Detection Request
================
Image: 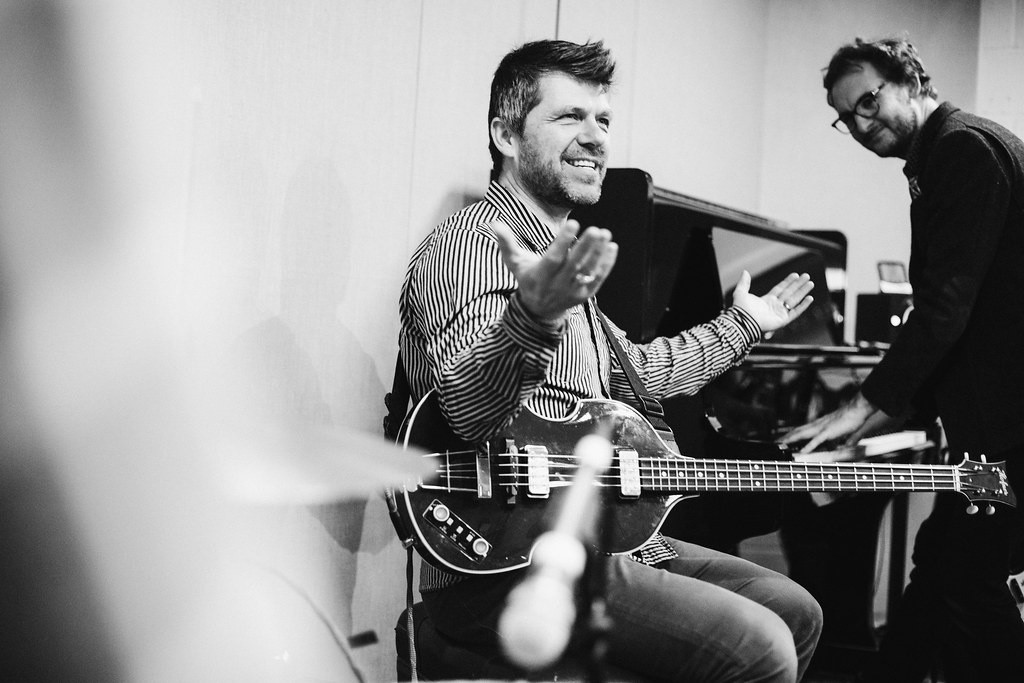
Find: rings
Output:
[575,273,597,285]
[784,302,790,310]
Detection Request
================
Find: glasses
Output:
[831,80,890,135]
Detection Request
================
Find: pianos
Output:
[461,164,946,654]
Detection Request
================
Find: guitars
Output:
[384,383,1019,586]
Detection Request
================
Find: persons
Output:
[772,36,1023,683]
[381,40,824,683]
